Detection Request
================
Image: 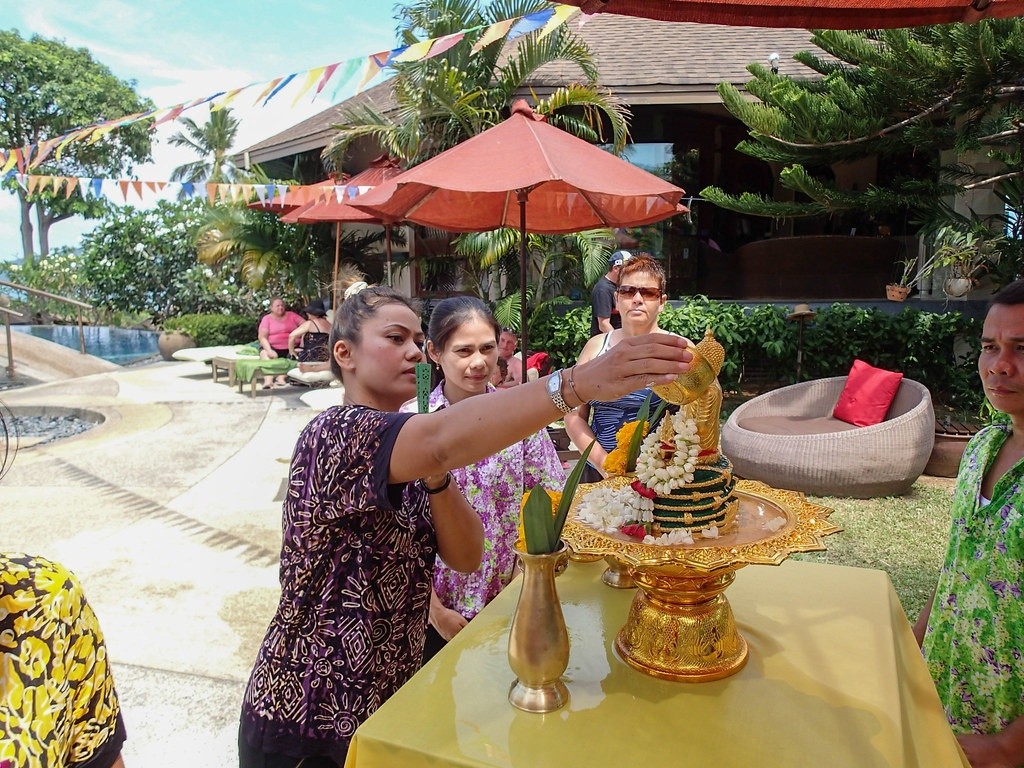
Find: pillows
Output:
[834,358,904,427]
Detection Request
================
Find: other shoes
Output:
[291,381,305,386]
[262,383,273,389]
[276,377,286,386]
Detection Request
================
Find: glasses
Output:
[617,285,662,301]
[502,327,517,335]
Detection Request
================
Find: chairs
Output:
[175,345,550,411]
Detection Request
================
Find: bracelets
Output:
[568,364,588,405]
[420,473,451,494]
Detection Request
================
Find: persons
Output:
[564,253,697,484]
[239,257,694,768]
[422,293,566,668]
[0,397,128,767]
[591,250,633,338]
[911,280,1024,768]
[257,295,307,388]
[490,357,509,387]
[679,322,723,452]
[287,297,333,385]
[498,329,518,360]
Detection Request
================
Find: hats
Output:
[608,251,632,267]
[303,300,327,316]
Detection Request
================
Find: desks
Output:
[344,560,973,768]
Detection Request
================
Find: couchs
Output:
[720,374,936,499]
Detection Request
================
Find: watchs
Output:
[545,367,577,415]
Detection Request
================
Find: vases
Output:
[509,537,571,714]
[599,555,637,588]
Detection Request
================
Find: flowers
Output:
[602,392,669,475]
[508,434,597,553]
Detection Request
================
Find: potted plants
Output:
[886,245,976,301]
[944,227,985,299]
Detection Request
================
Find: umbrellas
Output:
[345,96,691,382]
[247,172,353,352]
[275,150,406,287]
